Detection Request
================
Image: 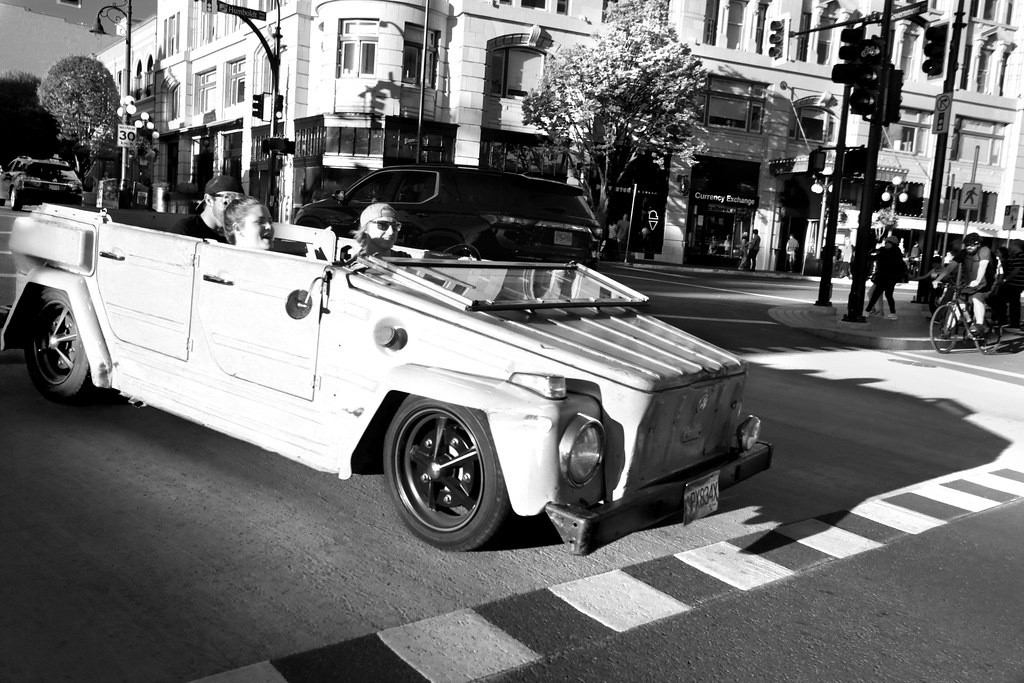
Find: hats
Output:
[360,203,399,229]
[883,236,899,246]
[642,228,650,233]
[196,175,245,210]
[961,233,980,244]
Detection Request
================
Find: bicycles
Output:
[928,283,1002,356]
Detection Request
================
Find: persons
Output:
[345,202,412,268]
[785,234,799,273]
[311,185,326,202]
[862,236,904,320]
[912,233,1024,340]
[737,230,760,272]
[608,214,650,262]
[709,236,718,254]
[172,175,245,245]
[221,194,275,251]
[724,235,732,255]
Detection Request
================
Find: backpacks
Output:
[990,253,1004,295]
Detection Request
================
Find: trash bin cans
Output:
[130,181,149,209]
[153,182,169,213]
[96,178,118,210]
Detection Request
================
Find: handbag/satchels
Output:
[897,261,909,283]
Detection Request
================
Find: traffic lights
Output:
[808,150,826,171]
[252,93,271,122]
[832,25,903,126]
[922,21,949,81]
[767,13,792,66]
[274,96,283,120]
[268,138,295,156]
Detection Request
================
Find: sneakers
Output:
[883,313,898,320]
[862,311,870,317]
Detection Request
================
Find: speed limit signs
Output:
[117,123,136,149]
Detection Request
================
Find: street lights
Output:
[811,167,834,270]
[880,176,909,237]
[89,0,136,191]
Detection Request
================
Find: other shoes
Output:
[870,309,883,316]
[926,316,941,324]
[970,324,986,338]
[937,331,951,339]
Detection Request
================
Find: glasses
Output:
[885,241,890,244]
[370,220,402,231]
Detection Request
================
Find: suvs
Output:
[293,164,603,274]
[0,156,82,211]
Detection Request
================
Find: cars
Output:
[0,203,776,556]
